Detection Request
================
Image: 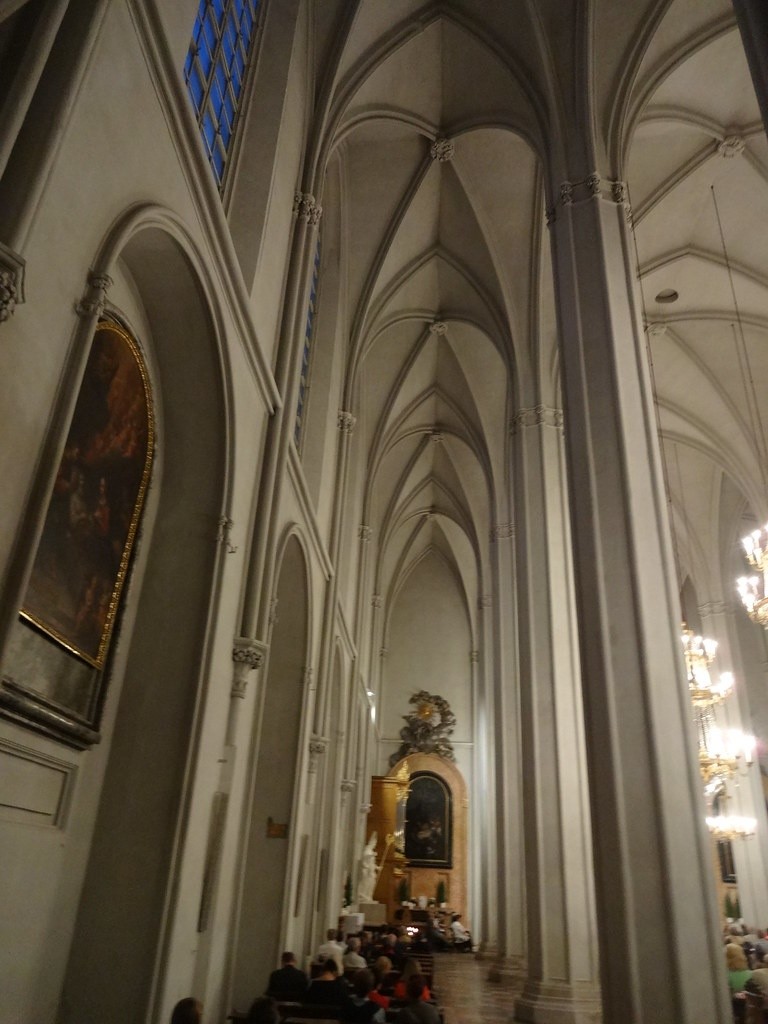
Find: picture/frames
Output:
[17,321,158,669]
[403,776,449,863]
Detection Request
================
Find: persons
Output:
[722,921,768,1024]
[453,914,473,952]
[153,923,441,1024]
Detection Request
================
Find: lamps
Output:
[737,521,768,630]
[681,621,758,844]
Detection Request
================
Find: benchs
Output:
[227,943,445,1024]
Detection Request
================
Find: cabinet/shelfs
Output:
[391,904,448,928]
